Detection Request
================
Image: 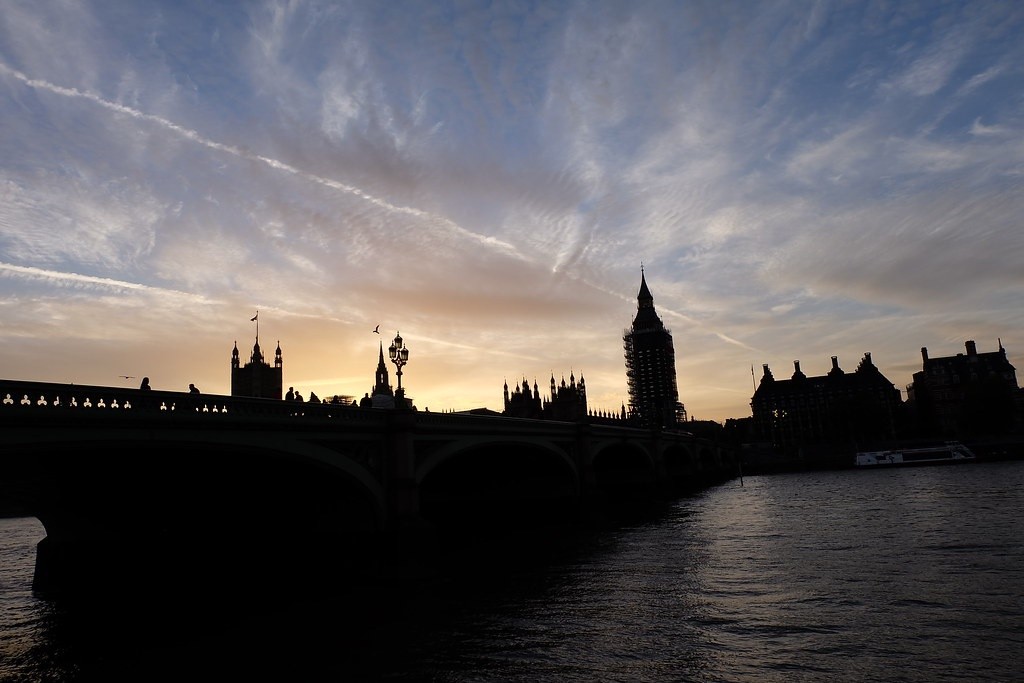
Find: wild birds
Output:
[119,376,134,379]
[373,324,379,334]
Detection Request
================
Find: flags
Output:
[250,315,258,321]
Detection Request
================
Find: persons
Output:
[322,399,328,404]
[285,387,303,416]
[360,393,373,407]
[351,400,358,406]
[330,396,340,404]
[425,407,429,412]
[141,377,151,389]
[190,383,200,394]
[413,406,418,411]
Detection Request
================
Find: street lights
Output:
[389,333,409,401]
